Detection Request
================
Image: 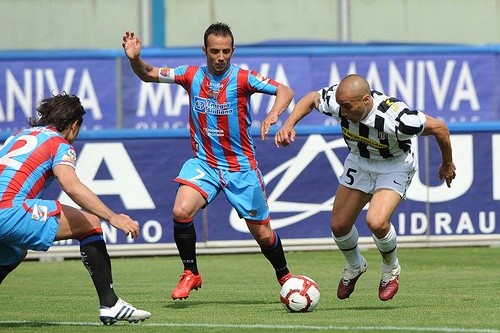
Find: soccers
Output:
[280,275,320,313]
